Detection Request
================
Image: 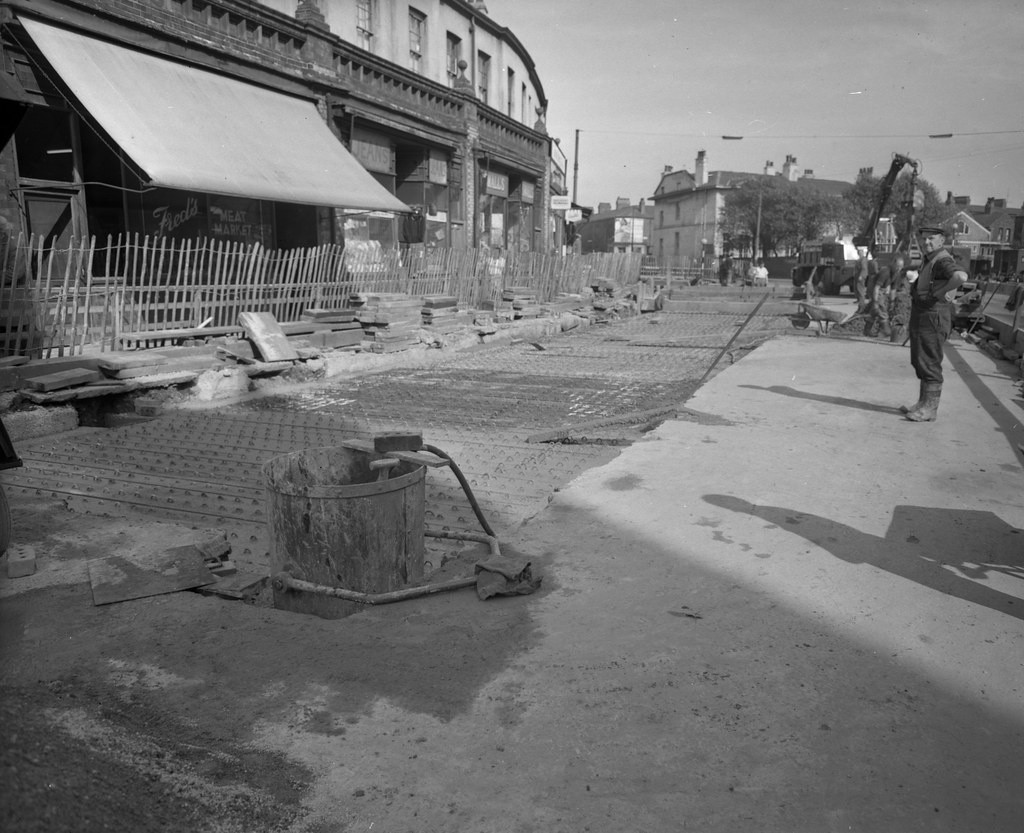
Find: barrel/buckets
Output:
[261,445,427,620]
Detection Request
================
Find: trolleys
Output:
[789,301,871,336]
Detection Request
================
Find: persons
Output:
[486,247,506,275]
[863,257,905,337]
[900,225,968,422]
[752,261,769,287]
[719,252,735,286]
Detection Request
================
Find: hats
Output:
[918,225,948,236]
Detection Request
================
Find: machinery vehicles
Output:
[853,153,986,332]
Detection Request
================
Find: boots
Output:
[906,382,942,422]
[880,321,892,336]
[900,380,924,412]
[863,321,878,336]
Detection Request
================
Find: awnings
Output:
[17,13,414,214]
